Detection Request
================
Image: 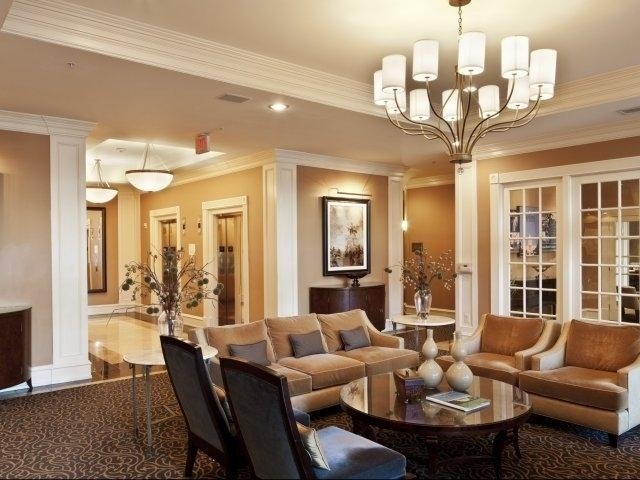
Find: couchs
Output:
[187,308,421,428]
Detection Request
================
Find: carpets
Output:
[0,370,640,479]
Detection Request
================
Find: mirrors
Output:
[85,206,108,294]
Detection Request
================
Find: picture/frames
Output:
[319,191,374,279]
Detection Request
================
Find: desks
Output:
[336,370,536,480]
[390,315,455,356]
[121,341,220,458]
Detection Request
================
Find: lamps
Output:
[82,155,120,209]
[124,134,175,195]
[370,0,560,175]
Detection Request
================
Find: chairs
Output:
[160,332,312,479]
[437,309,554,424]
[213,353,408,479]
[614,282,639,322]
[517,317,640,451]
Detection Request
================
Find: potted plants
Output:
[377,243,463,320]
[102,242,231,344]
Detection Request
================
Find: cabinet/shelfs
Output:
[508,277,559,318]
[307,282,388,332]
[0,301,38,399]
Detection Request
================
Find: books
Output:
[425,390,492,411]
[426,400,490,417]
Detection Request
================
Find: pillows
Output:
[287,329,328,359]
[335,326,374,350]
[225,341,276,369]
[292,420,331,470]
[212,381,237,423]
